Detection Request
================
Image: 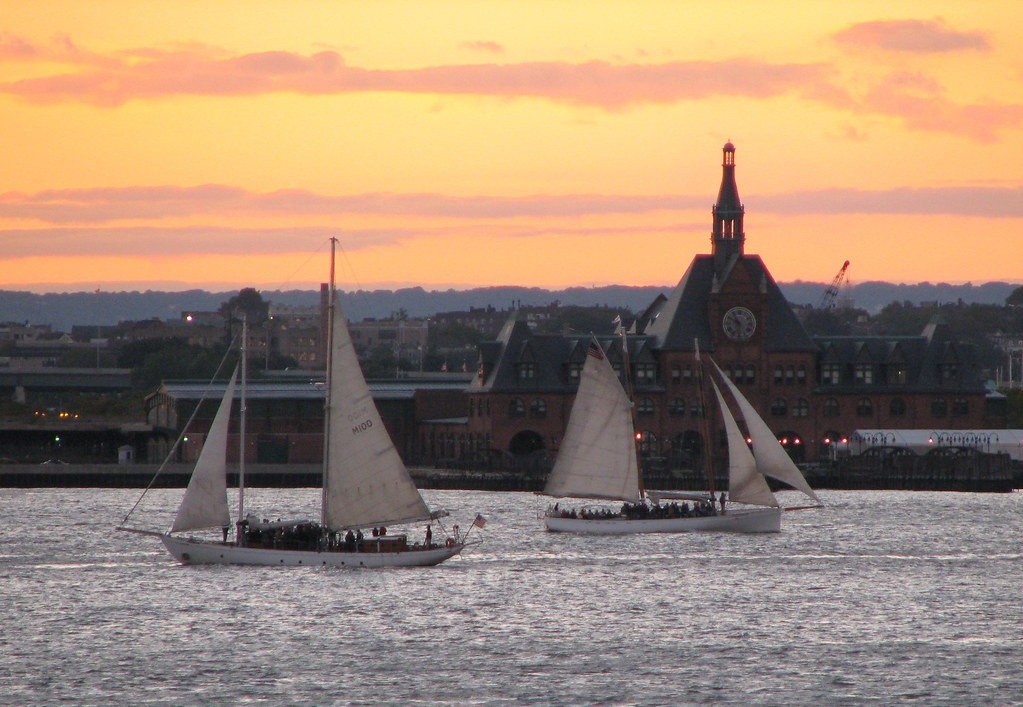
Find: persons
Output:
[553,492,726,520]
[222,518,433,552]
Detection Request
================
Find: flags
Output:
[474,515,486,529]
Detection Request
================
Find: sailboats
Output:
[533,327,826,534]
[115,237,483,567]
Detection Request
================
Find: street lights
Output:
[863,432,882,447]
[876,433,896,446]
[824,433,847,460]
[851,432,870,454]
[929,432,999,452]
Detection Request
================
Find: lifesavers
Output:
[446,538,452,548]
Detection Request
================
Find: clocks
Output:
[723,306,756,341]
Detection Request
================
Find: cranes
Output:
[818,260,850,309]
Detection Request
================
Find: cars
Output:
[925,446,980,457]
[844,446,919,458]
